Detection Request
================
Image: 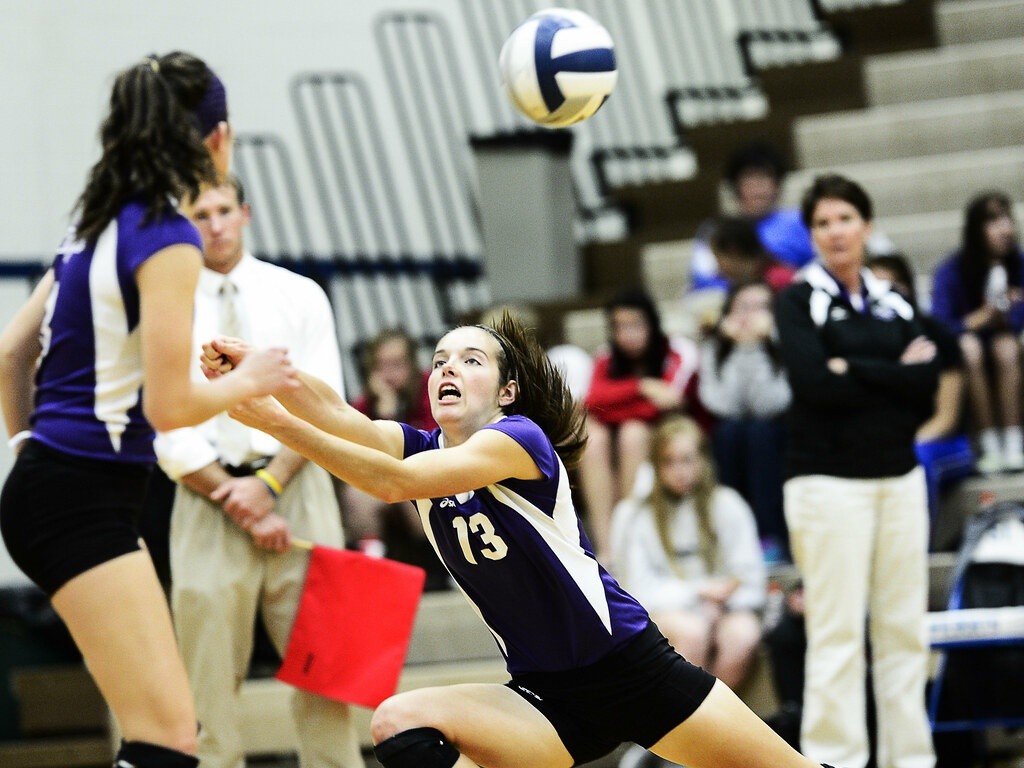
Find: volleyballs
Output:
[497,7,620,130]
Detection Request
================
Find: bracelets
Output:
[256,469,285,501]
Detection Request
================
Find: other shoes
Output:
[977,450,1024,474]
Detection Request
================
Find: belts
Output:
[223,456,272,477]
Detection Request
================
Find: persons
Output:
[200,155,1024,768]
[771,176,966,768]
[1,54,369,767]
[203,323,846,768]
[152,169,360,768]
